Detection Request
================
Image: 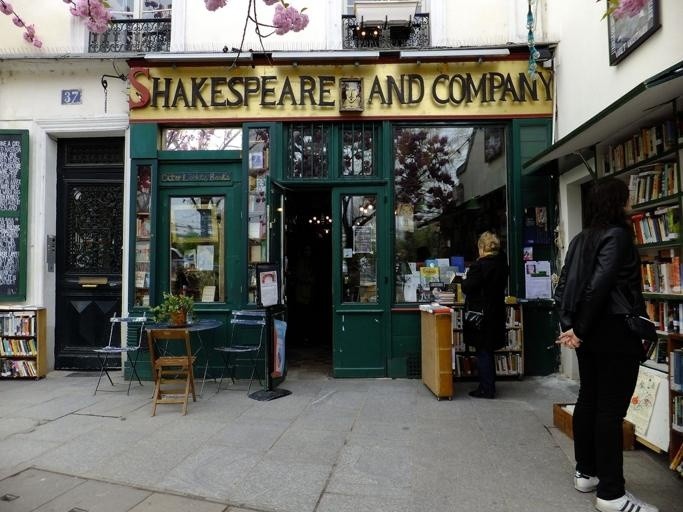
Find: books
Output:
[0,309,37,377]
[596,114,682,446]
[133,149,278,309]
[417,288,524,382]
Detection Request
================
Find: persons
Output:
[459,230,510,399]
[552,176,662,512]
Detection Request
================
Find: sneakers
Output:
[469,384,495,399]
[573,470,602,493]
[594,490,659,512]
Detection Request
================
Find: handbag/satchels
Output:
[464,310,484,331]
[619,312,658,363]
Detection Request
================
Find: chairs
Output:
[92,310,269,417]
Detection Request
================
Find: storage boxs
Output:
[553,402,636,451]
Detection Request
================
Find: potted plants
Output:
[147,290,196,326]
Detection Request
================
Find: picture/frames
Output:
[606,0,662,67]
[169,203,219,244]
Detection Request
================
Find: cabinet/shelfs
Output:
[0,308,46,381]
[598,146,682,374]
[450,300,526,382]
